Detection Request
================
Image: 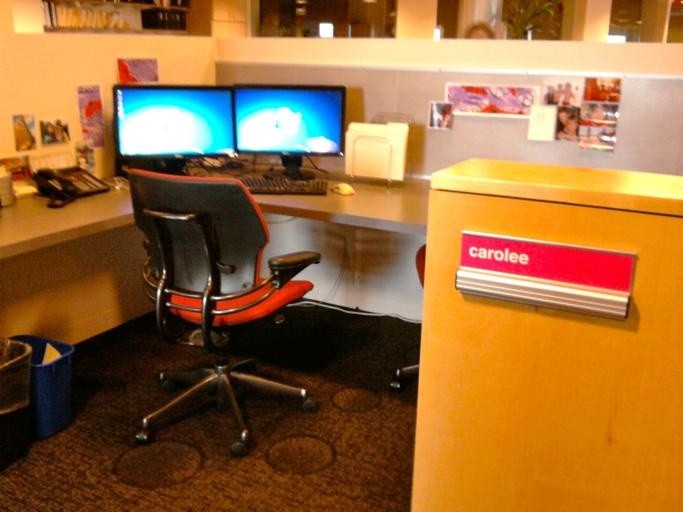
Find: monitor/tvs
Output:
[113,84,235,176]
[608,34,627,44]
[435,25,444,38]
[232,83,346,180]
[319,23,333,38]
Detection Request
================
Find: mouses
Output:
[330,183,355,196]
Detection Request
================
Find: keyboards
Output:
[235,176,327,195]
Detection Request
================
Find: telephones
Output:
[32,167,110,201]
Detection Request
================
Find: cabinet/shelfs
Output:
[410,158,682,511]
[43,1,681,80]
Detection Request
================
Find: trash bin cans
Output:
[0,334,74,472]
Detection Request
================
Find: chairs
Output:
[128,168,321,454]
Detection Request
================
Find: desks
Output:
[0,162,430,511]
